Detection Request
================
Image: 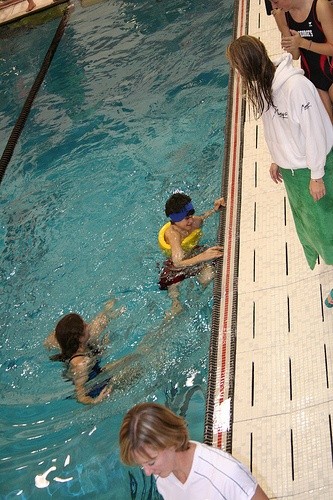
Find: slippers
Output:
[325,289,333,308]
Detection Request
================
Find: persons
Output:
[270,0,332,120]
[120,403,270,500]
[26,0,36,11]
[158,192,226,323]
[45,300,139,405]
[226,35,333,308]
